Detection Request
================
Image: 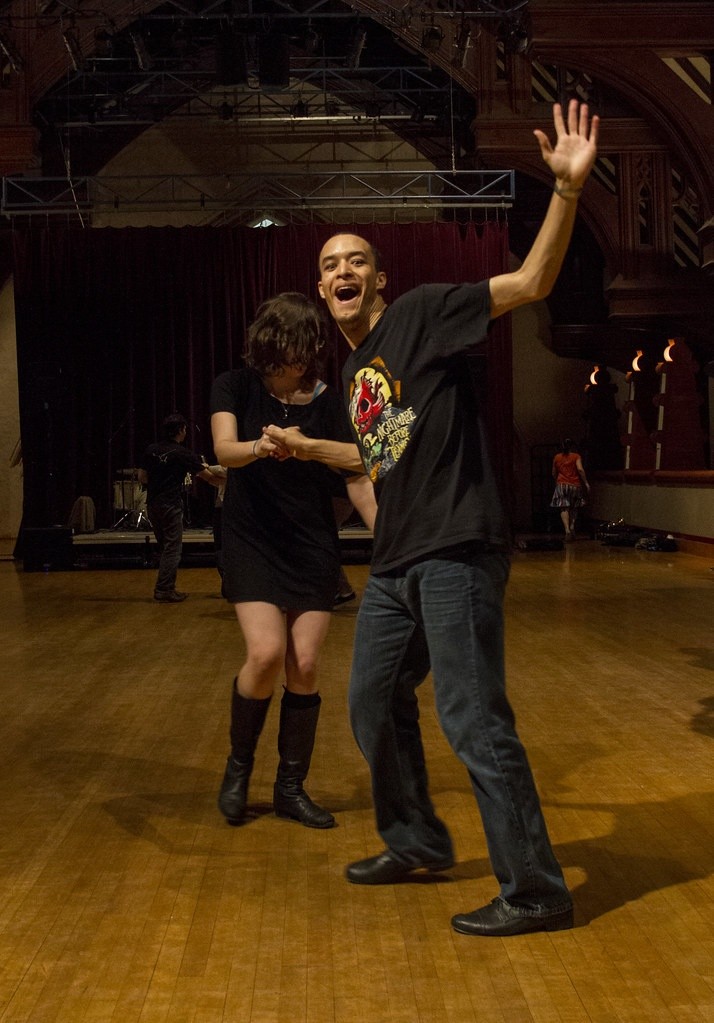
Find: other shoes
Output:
[570,527,576,539]
[154,589,186,602]
[564,533,572,541]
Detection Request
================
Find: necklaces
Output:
[275,390,297,417]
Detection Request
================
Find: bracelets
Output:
[253,440,261,459]
[554,183,583,199]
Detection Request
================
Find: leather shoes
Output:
[449,897,575,936]
[346,850,456,884]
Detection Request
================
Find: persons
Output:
[209,292,378,828]
[262,98,601,936]
[552,438,590,542]
[138,415,228,601]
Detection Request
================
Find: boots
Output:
[274,684,335,828]
[219,675,274,821]
